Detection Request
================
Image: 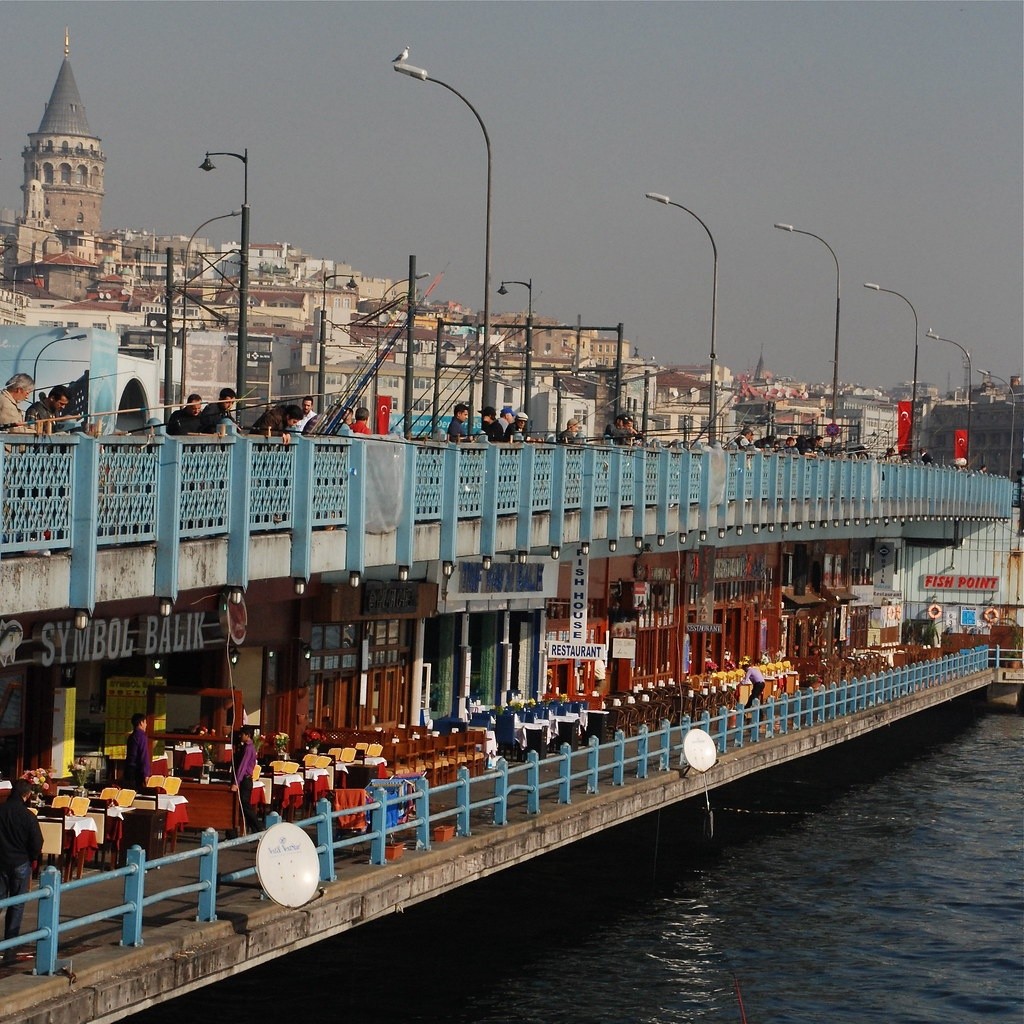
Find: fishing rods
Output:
[1,392,325,432]
[308,256,457,436]
[385,288,543,440]
[126,388,364,435]
[411,376,1001,472]
[237,423,480,456]
[32,370,137,391]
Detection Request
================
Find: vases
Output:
[708,671,711,675]
[433,826,456,843]
[812,682,820,690]
[206,759,214,766]
[74,786,87,798]
[310,748,317,755]
[277,749,284,755]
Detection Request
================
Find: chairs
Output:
[841,652,889,684]
[693,661,795,691]
[177,743,384,784]
[420,701,587,761]
[380,725,486,787]
[603,683,736,741]
[27,775,182,817]
[164,742,175,776]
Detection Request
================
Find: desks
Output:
[423,709,430,726]
[0,780,13,805]
[700,670,800,707]
[470,705,486,717]
[30,791,190,883]
[193,756,387,823]
[464,710,609,763]
[166,744,233,771]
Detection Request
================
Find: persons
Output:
[734,428,754,449]
[497,408,516,435]
[795,435,830,459]
[477,406,503,441]
[594,660,606,697]
[447,403,474,442]
[884,447,933,465]
[167,394,216,435]
[601,414,646,446]
[249,403,303,522]
[741,664,766,722]
[978,464,986,473]
[706,639,712,658]
[226,683,248,726]
[223,725,265,844]
[849,446,868,460]
[342,408,372,435]
[755,434,794,452]
[0,372,72,557]
[0,778,45,963]
[125,713,152,790]
[295,396,318,436]
[200,387,241,433]
[558,416,587,443]
[504,411,532,442]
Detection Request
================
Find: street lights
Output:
[926,333,972,468]
[497,279,534,437]
[645,192,718,445]
[317,272,359,415]
[863,283,918,459]
[198,148,251,428]
[33,334,88,403]
[180,209,243,410]
[373,272,430,434]
[773,222,840,453]
[977,369,1015,479]
[393,63,492,409]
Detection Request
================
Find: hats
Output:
[617,414,630,420]
[477,407,495,415]
[500,408,515,417]
[516,412,528,421]
[568,419,578,425]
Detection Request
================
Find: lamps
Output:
[229,647,241,670]
[60,663,76,684]
[296,638,314,663]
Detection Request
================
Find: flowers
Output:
[252,729,327,756]
[806,671,823,684]
[510,692,568,713]
[19,756,95,794]
[190,722,216,760]
[705,654,769,672]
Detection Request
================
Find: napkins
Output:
[105,798,119,807]
[491,716,496,724]
[156,786,167,794]
[62,806,74,817]
[514,713,519,728]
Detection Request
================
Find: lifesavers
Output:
[928,604,942,619]
[888,606,895,620]
[896,605,901,620]
[983,608,999,623]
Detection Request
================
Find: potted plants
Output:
[1003,624,1024,669]
[386,833,405,861]
